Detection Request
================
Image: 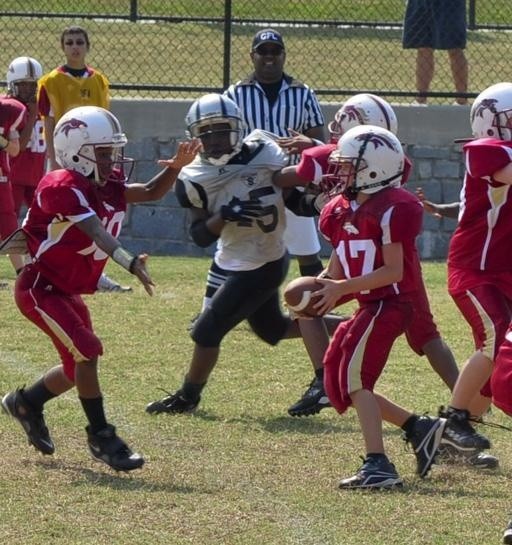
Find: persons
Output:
[2,105,203,472]
[0,93,31,288]
[35,26,132,291]
[144,91,356,418]
[269,80,512,490]
[3,55,55,263]
[400,0,471,108]
[188,29,328,327]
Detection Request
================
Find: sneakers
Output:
[85,424,145,472]
[435,406,492,453]
[144,387,204,416]
[401,412,447,479]
[434,446,500,469]
[1,383,55,455]
[287,376,333,417]
[338,455,406,490]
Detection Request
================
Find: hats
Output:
[251,29,286,51]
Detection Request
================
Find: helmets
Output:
[53,106,129,187]
[328,124,405,193]
[328,92,397,139]
[184,93,248,171]
[470,82,512,142]
[7,56,43,104]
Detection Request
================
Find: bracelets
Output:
[111,245,138,272]
[2,140,9,152]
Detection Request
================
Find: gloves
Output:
[220,196,266,227]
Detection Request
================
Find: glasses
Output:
[63,38,86,45]
[248,46,288,56]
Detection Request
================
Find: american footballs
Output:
[284,276,333,318]
